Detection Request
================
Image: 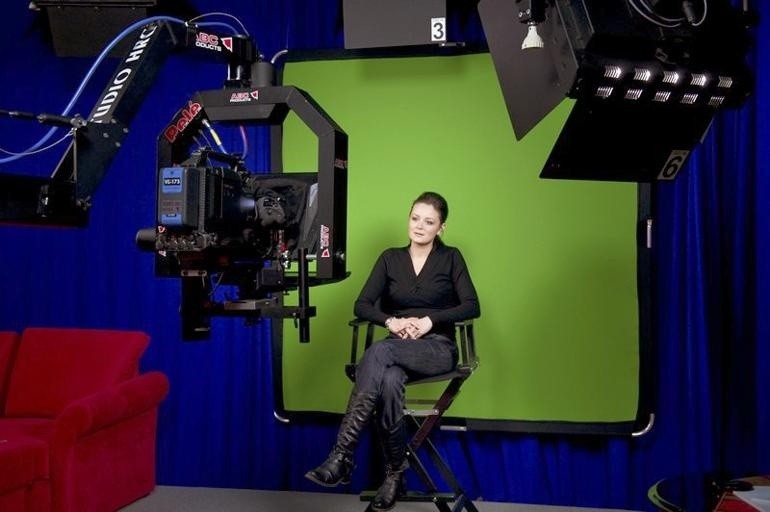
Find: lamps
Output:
[518,2,553,50]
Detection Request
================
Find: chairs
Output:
[2,327,168,512]
[346,317,481,511]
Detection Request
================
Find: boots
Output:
[371,416,407,511]
[304,388,379,487]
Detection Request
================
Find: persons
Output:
[301,191,482,511]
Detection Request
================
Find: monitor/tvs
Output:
[249,171,318,262]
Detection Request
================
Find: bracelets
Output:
[386,316,396,327]
